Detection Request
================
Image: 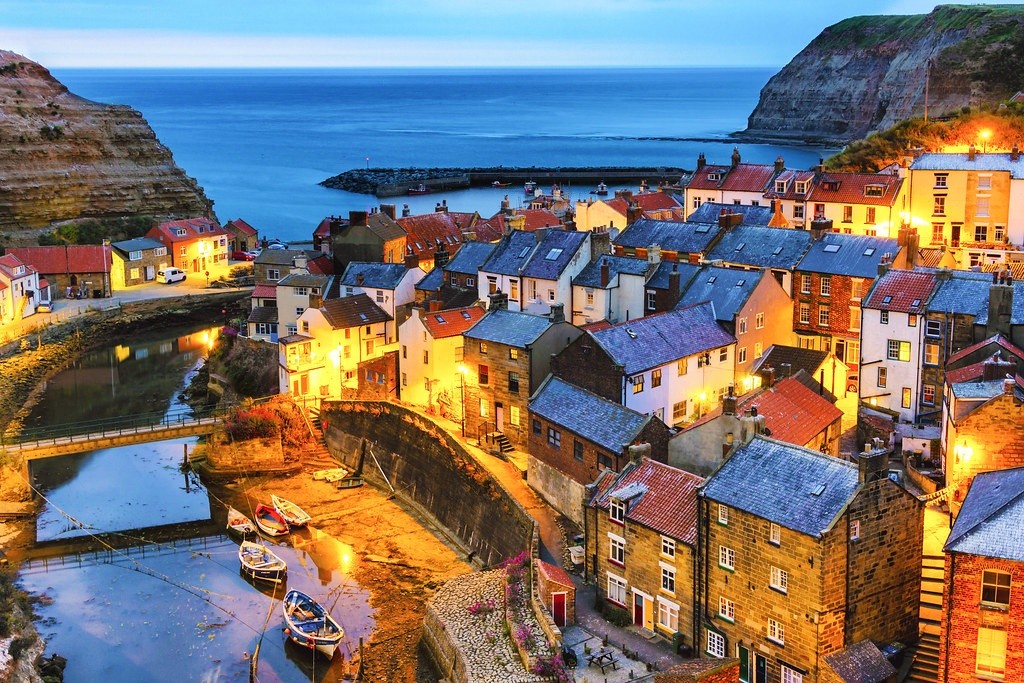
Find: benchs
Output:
[586,656,619,668]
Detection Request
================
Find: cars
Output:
[268,244,284,250]
[37,300,54,312]
[232,251,253,261]
[268,241,289,249]
[249,251,261,258]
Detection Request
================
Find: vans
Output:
[156,267,186,284]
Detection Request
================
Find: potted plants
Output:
[629,652,635,659]
[567,658,576,669]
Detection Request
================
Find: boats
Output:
[336,477,364,488]
[524,182,536,196]
[598,179,608,195]
[281,626,344,683]
[282,587,345,661]
[240,566,288,601]
[239,540,288,588]
[492,181,512,187]
[270,494,312,526]
[227,506,258,540]
[255,502,289,536]
[325,470,348,482]
[408,184,430,195]
[313,468,342,481]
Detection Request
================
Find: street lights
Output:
[460,366,466,438]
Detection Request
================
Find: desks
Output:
[590,647,616,674]
